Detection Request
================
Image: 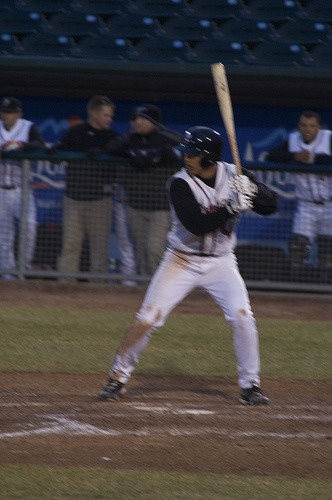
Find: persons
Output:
[0,94,332,285]
[93,126,278,405]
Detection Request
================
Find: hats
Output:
[135,103,161,125]
[0,97,23,113]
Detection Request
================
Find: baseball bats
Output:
[210,62,244,177]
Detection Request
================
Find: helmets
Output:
[174,126,221,169]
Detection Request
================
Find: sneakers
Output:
[239,386,268,405]
[97,379,127,401]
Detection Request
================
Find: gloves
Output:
[226,194,253,215]
[136,149,154,173]
[228,175,257,198]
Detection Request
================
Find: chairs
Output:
[0,0,332,70]
